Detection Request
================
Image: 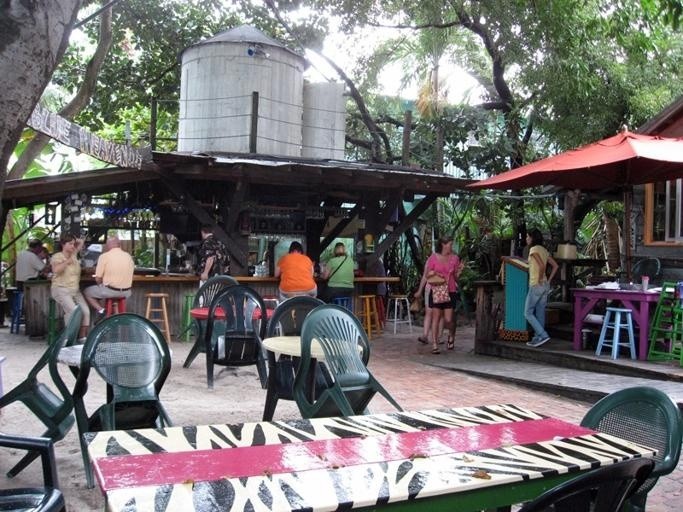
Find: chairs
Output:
[1,435,68,511]
[258,298,328,390]
[0,305,85,478]
[176,274,243,370]
[579,387,682,512]
[631,256,662,283]
[510,457,652,512]
[291,303,403,418]
[204,284,268,390]
[72,314,176,487]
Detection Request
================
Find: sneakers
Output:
[417,336,429,344]
[77,337,86,344]
[526,342,534,346]
[534,337,550,347]
[438,337,444,344]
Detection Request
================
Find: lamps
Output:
[338,199,358,211]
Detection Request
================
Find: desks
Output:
[183,307,275,374]
[82,403,660,512]
[500,255,532,342]
[258,336,364,420]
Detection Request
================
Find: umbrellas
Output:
[466,123,682,284]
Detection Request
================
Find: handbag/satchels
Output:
[410,297,423,319]
[433,255,451,304]
[318,256,348,292]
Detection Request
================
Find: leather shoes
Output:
[94,310,105,325]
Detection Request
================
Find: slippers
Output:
[431,348,440,354]
[448,335,454,350]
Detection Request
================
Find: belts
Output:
[105,285,131,292]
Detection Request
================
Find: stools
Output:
[647,306,682,365]
[46,297,66,347]
[10,291,27,334]
[356,294,381,341]
[593,306,637,364]
[649,282,683,345]
[140,292,171,345]
[386,295,413,336]
[333,297,352,311]
[102,298,126,319]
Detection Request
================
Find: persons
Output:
[413,253,446,345]
[426,236,466,355]
[274,241,317,303]
[37,247,49,266]
[319,242,355,305]
[522,227,558,348]
[14,239,49,291]
[355,241,387,296]
[83,236,134,324]
[49,234,90,345]
[194,224,231,309]
